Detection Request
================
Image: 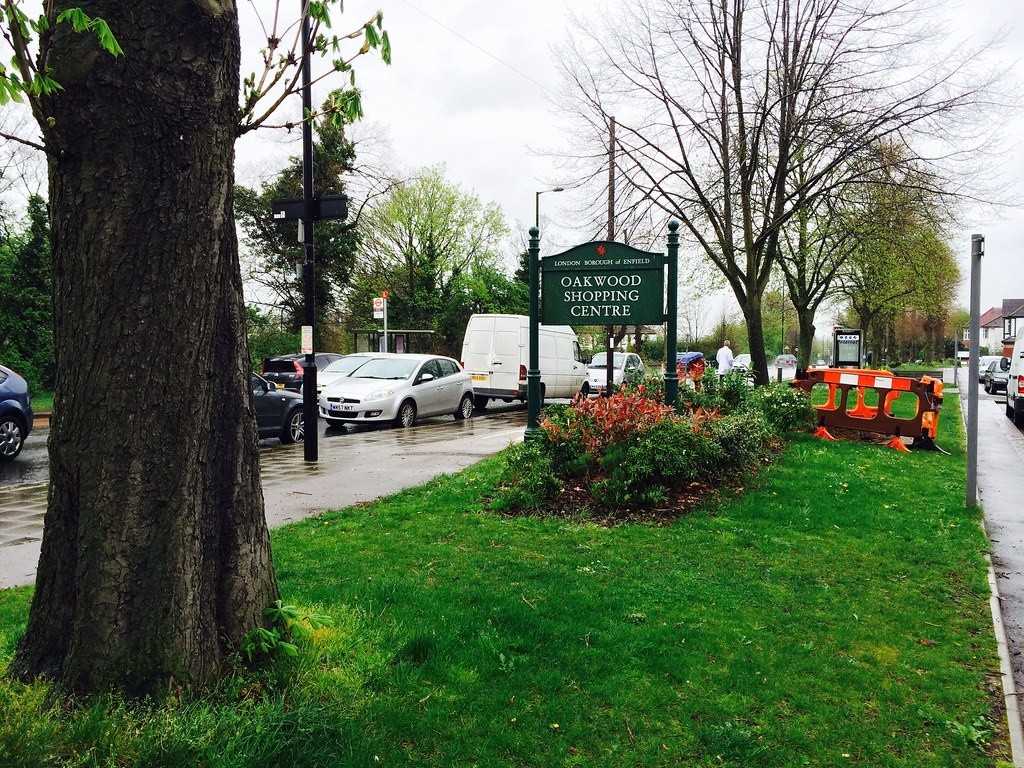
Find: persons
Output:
[687,357,708,391]
[716,340,734,384]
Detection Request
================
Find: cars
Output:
[251,371,306,444]
[733,353,753,377]
[261,351,345,393]
[318,352,475,430]
[0,364,35,464]
[300,352,391,406]
[979,356,1001,381]
[666,351,704,372]
[984,360,1010,394]
[589,352,646,396]
[817,360,825,366]
[775,355,799,369]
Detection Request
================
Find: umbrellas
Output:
[680,351,703,363]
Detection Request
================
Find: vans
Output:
[1000,326,1024,427]
[461,313,590,412]
